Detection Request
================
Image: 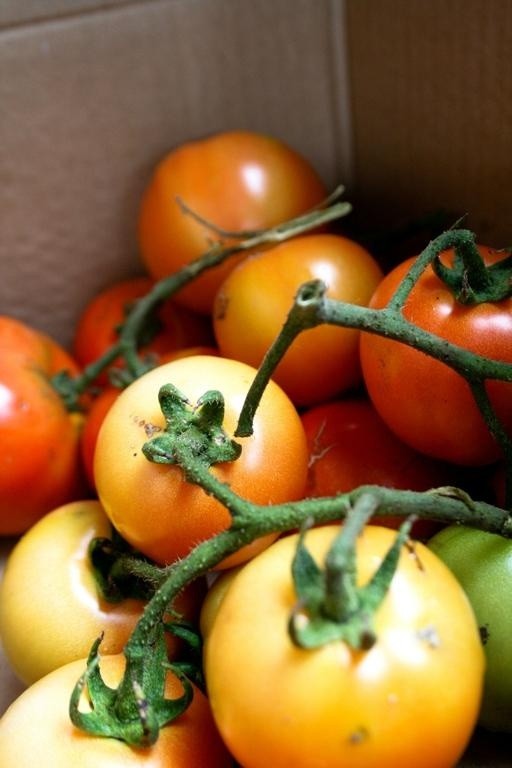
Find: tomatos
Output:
[0,129,512,768]
